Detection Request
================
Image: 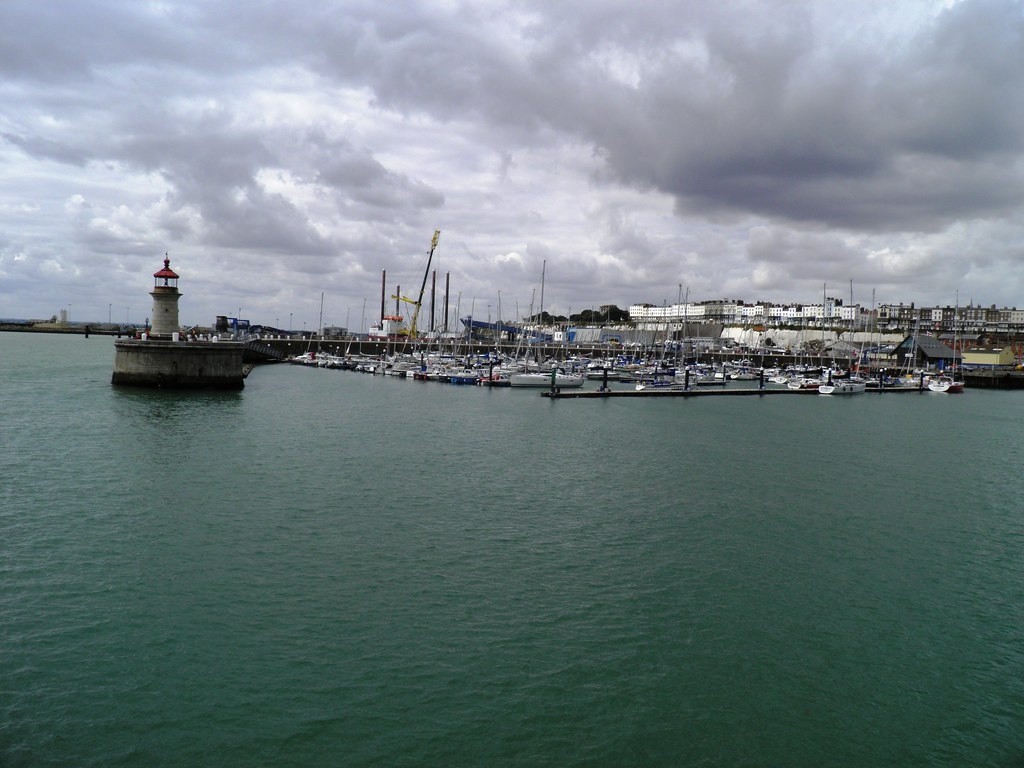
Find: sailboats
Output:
[286,258,967,395]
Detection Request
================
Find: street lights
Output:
[303,322,306,332]
[290,313,293,334]
[277,318,279,332]
[68,304,72,325]
[238,308,241,320]
[109,304,112,326]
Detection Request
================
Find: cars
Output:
[721,344,748,352]
[953,365,974,371]
[801,349,827,356]
[765,346,791,354]
